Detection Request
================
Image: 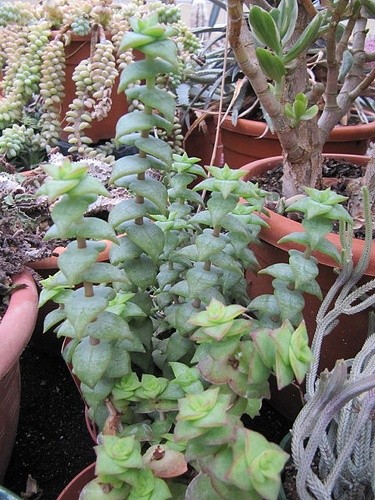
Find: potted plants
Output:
[1,0,375,500]
